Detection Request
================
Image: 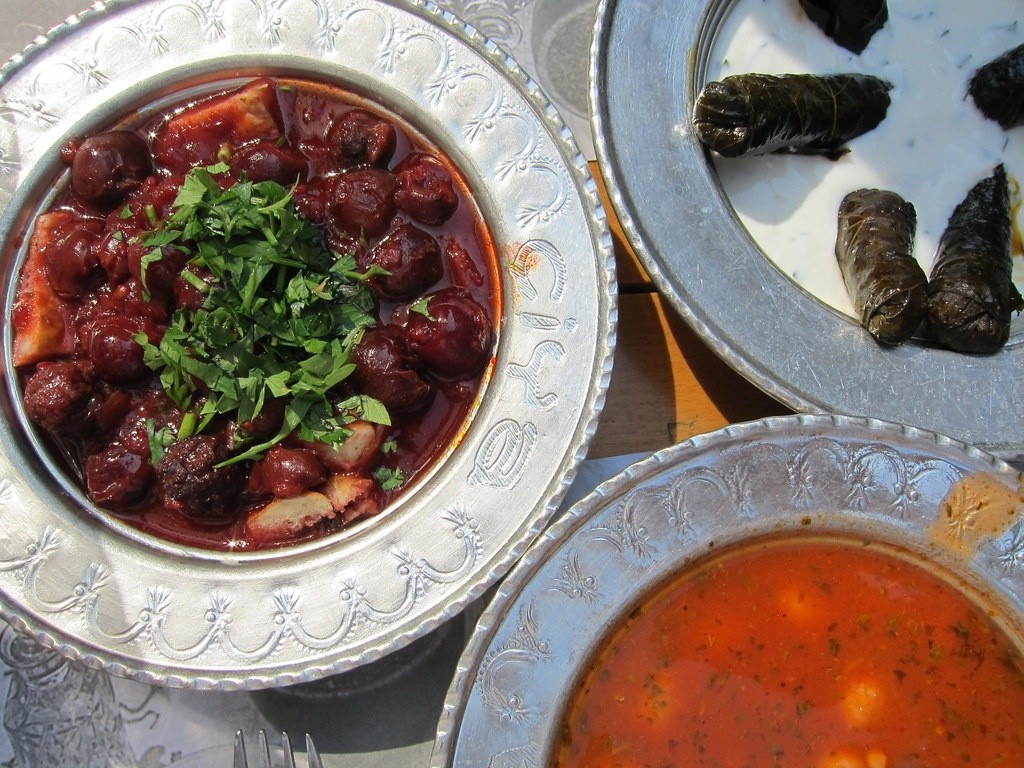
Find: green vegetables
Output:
[115,164,433,467]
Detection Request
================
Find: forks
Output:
[233,729,323,767]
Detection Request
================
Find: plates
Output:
[0,0,618,693]
[429,413,1022,767]
[587,0,1024,459]
